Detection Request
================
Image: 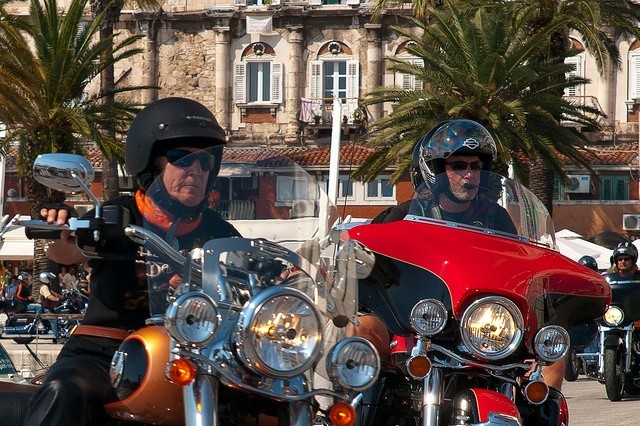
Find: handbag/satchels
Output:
[15,303,26,313]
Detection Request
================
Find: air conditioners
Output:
[74,204,94,217]
[623,215,640,231]
[564,175,590,194]
[291,200,315,218]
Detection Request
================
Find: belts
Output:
[71,323,133,340]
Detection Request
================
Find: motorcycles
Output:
[0,144,380,426]
[1,281,81,344]
[565,324,601,381]
[600,272,639,401]
[332,172,612,426]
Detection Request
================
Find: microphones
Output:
[463,182,492,194]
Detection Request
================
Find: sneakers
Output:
[36,321,45,329]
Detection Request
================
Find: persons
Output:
[59,266,68,288]
[6,275,18,299]
[208,187,221,209]
[609,241,640,281]
[37,273,64,340]
[16,272,42,323]
[326,118,567,426]
[42,97,317,426]
[77,271,90,296]
[63,267,76,290]
[0,289,5,311]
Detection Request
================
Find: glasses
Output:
[159,147,216,170]
[617,256,631,260]
[445,161,485,171]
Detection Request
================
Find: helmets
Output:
[78,271,88,280]
[410,118,497,195]
[18,272,32,284]
[38,271,56,283]
[124,97,226,177]
[613,240,637,260]
